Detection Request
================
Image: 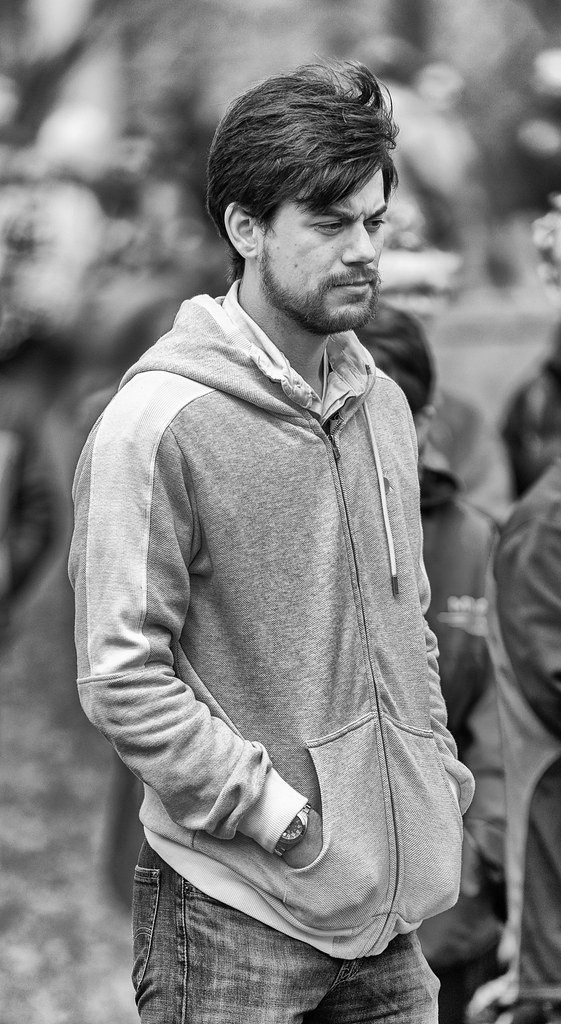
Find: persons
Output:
[68,64,476,1024]
[332,298,511,1023]
[0,312,80,644]
[481,427,561,1024]
[489,213,561,507]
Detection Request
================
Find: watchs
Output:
[273,800,313,856]
